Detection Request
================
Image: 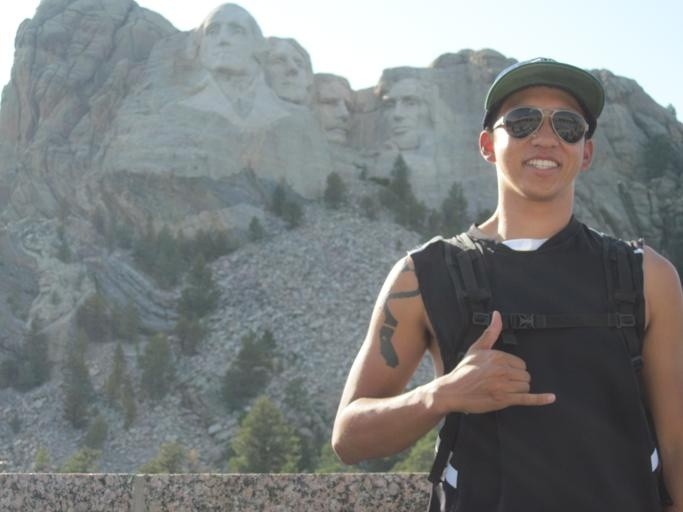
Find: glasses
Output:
[492,106,591,144]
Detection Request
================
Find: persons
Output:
[190,0,267,79]
[255,30,317,105]
[310,68,359,148]
[371,63,444,154]
[327,56,682,512]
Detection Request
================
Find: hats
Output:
[481,55,604,120]
